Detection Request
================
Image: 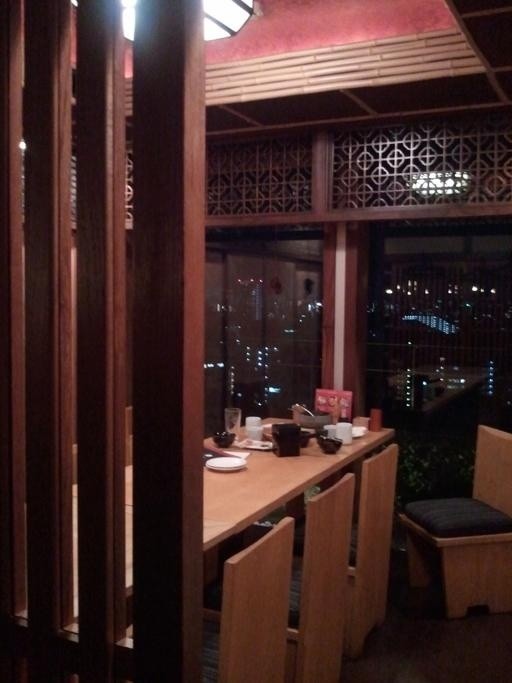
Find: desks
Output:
[71,416,397,634]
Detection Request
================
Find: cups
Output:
[301,430,311,449]
[245,415,264,440]
[224,406,242,438]
[335,422,353,444]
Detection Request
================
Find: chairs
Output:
[129,472,296,518]
[73,406,135,487]
[398,422,512,619]
[290,449,398,663]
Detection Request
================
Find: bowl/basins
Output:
[317,437,343,453]
[297,410,332,429]
[212,430,236,448]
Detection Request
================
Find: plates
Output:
[205,456,247,472]
[323,424,369,438]
[236,438,274,451]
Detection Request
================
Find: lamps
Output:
[71,0,255,46]
